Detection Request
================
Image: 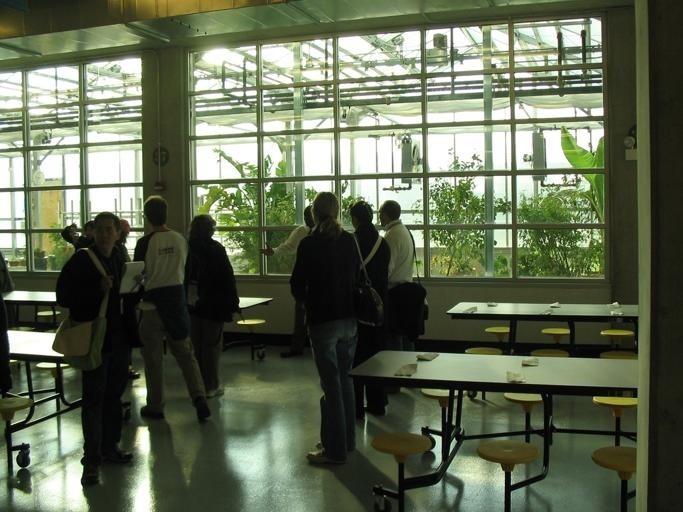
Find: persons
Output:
[350,201,391,419]
[379,200,416,394]
[260,206,317,357]
[134,195,210,419]
[188,214,238,398]
[56,210,143,485]
[289,191,370,464]
[0,251,15,396]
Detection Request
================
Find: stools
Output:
[499,393,552,449]
[597,348,638,366]
[419,388,471,450]
[540,326,571,344]
[599,327,635,345]
[363,430,449,510]
[461,344,505,400]
[478,432,554,510]
[0,287,283,477]
[523,350,568,366]
[588,437,638,510]
[589,395,644,448]
[482,325,511,349]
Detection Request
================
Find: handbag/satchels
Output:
[356,287,384,324]
[387,282,429,336]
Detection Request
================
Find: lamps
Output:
[621,135,638,162]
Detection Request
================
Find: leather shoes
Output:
[128,369,140,377]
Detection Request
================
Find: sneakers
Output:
[206,386,225,397]
[108,451,132,463]
[52,317,107,369]
[81,465,99,487]
[194,396,210,422]
[140,405,167,419]
[307,441,347,464]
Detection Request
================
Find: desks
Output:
[346,348,640,505]
[445,299,639,355]
